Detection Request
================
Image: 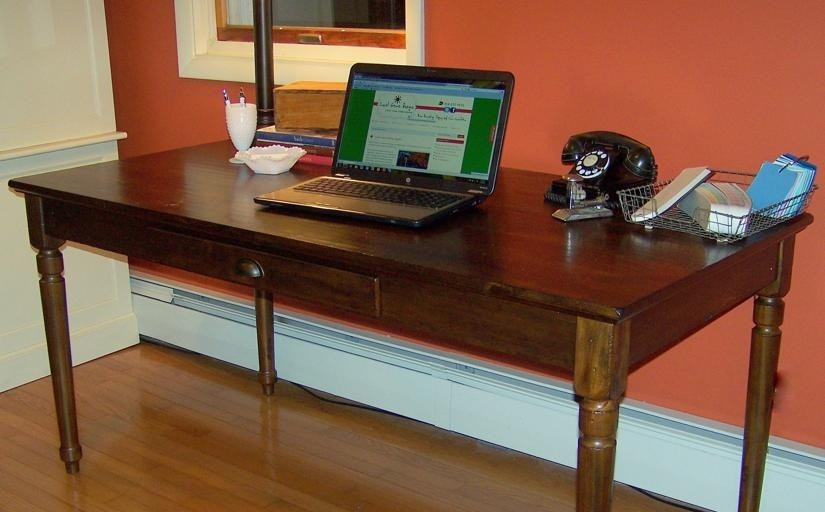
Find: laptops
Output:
[253,63,516,228]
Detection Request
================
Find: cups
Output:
[225,102,258,165]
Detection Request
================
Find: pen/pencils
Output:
[221,89,232,109]
[240,88,247,109]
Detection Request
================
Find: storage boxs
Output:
[274,80,348,131]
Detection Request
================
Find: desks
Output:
[9,133,814,512]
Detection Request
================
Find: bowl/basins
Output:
[234,144,308,175]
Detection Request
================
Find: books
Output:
[747,153,816,220]
[631,166,715,221]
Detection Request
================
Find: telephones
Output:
[543,131,656,209]
